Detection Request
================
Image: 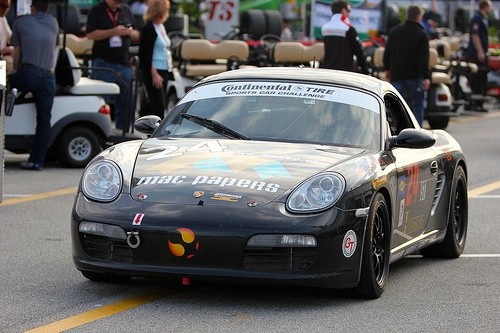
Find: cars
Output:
[0,32,500,169]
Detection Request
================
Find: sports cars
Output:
[66,59,471,301]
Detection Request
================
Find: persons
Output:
[383,5,431,129]
[465,0,493,114]
[280,18,292,67]
[310,102,372,148]
[0,0,13,119]
[139,0,172,138]
[85,0,140,144]
[5,0,60,169]
[321,0,369,75]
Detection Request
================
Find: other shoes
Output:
[471,104,487,111]
[19,162,43,170]
